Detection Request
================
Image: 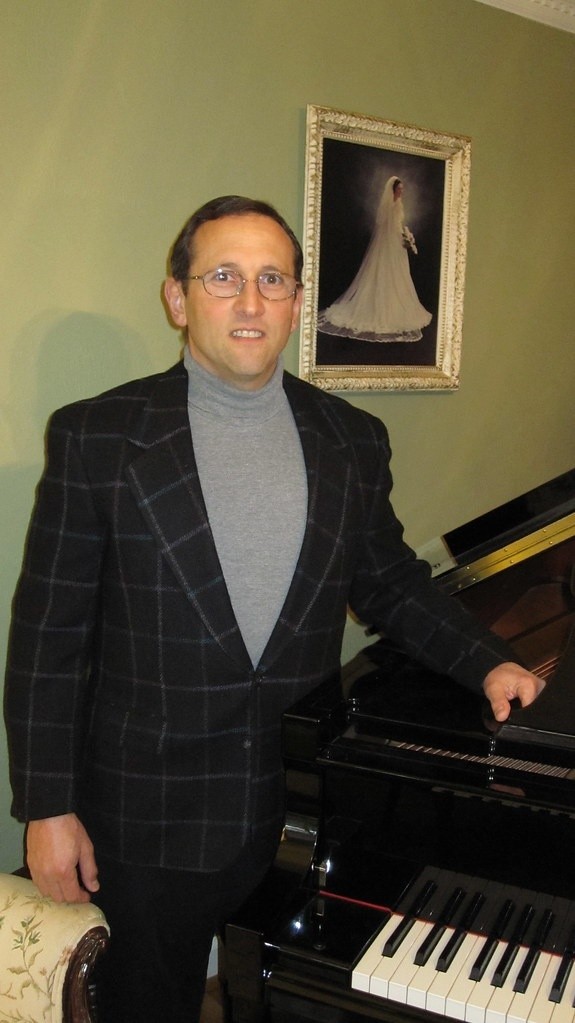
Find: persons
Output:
[315,176,433,342]
[3,196,545,1023]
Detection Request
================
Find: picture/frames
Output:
[300,102,471,393]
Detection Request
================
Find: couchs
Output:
[0,872,110,1023]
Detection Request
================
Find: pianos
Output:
[212,463,574,1023]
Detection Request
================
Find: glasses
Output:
[179,267,302,301]
